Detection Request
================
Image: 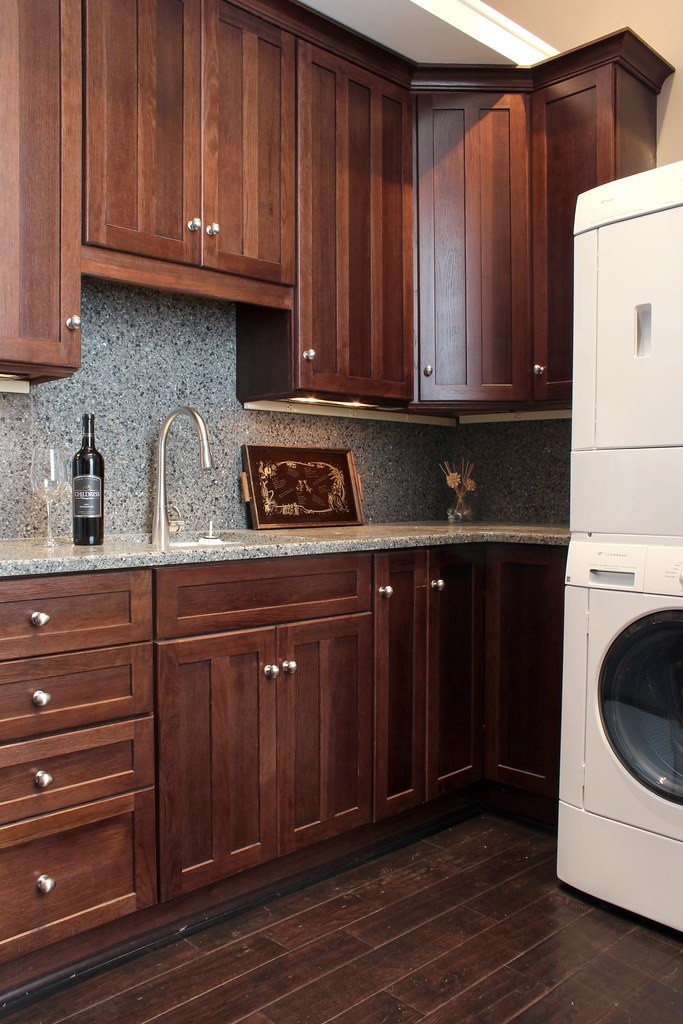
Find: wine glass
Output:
[29,447,71,550]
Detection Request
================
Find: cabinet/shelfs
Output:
[292,4,415,401]
[486,539,568,822]
[560,168,683,595]
[375,543,487,824]
[0,567,161,1010]
[0,0,84,380]
[531,20,677,404]
[152,553,375,894]
[418,61,532,415]
[84,1,300,312]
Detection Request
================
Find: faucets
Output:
[152,406,214,552]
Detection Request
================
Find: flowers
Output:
[440,459,478,494]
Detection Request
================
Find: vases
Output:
[447,495,474,522]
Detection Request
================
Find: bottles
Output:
[71,412,106,546]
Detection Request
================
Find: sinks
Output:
[117,529,320,548]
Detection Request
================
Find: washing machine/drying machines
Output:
[554,156,683,931]
